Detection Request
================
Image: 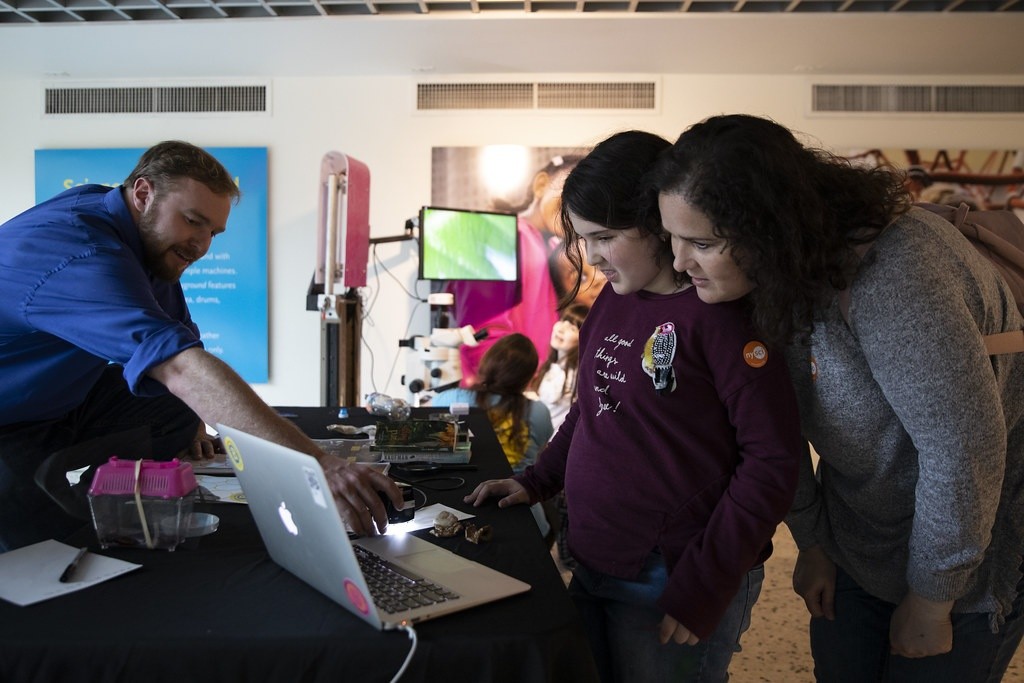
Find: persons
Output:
[422,332,576,570]
[462,129,797,683]
[442,152,607,390]
[531,302,590,442]
[640,111,1024,683]
[0,141,407,535]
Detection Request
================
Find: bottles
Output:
[364,392,410,421]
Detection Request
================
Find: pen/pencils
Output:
[59,547,87,583]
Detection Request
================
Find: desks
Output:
[0,407,606,683]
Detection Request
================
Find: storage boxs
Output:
[86,456,199,553]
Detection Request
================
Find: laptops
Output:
[218,421,534,629]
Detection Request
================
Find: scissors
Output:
[397,460,478,471]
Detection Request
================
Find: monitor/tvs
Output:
[420,205,521,282]
